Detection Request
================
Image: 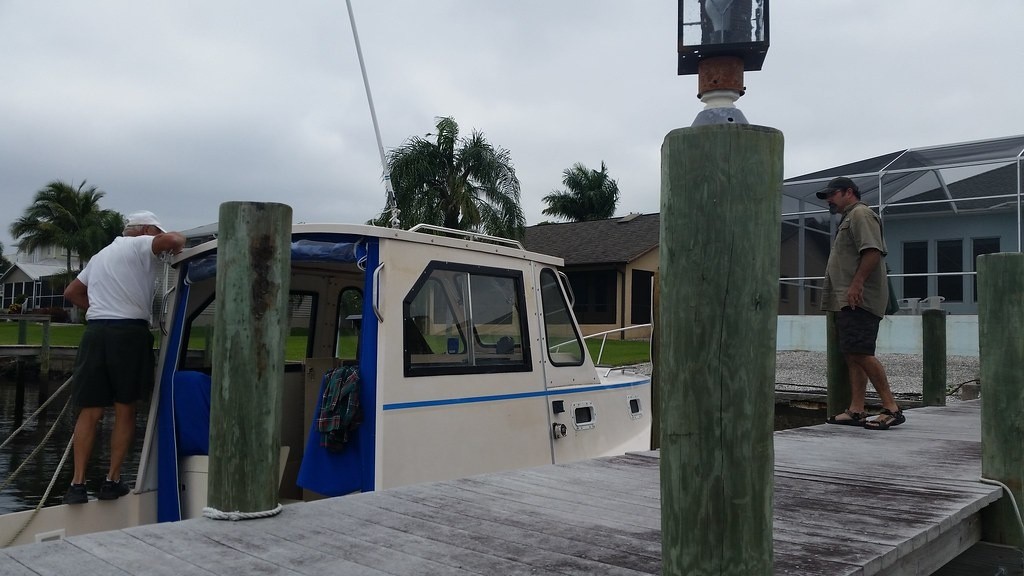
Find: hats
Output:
[816,177,859,200]
[127,210,167,234]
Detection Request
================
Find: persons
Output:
[62,210,185,505]
[816,177,906,429]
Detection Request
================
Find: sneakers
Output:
[62,482,88,505]
[98,480,130,500]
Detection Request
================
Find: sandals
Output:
[864,408,906,430]
[824,408,866,427]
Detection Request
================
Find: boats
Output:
[0,0,653,549]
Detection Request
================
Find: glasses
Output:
[828,190,841,197]
